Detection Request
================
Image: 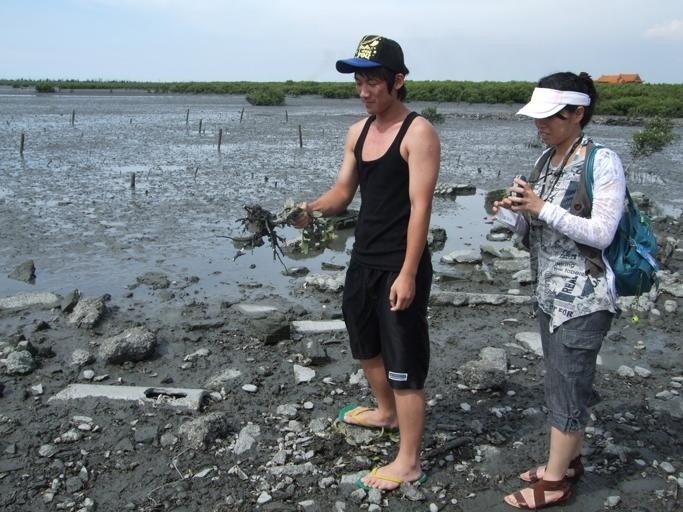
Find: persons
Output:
[492,70,628,510]
[288,33,445,490]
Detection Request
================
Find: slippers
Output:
[338,405,398,433]
[355,466,427,495]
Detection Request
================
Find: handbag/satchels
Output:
[583,145,659,326]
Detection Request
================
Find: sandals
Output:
[502,475,575,511]
[515,454,582,484]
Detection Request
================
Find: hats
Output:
[514,87,590,119]
[335,34,403,73]
